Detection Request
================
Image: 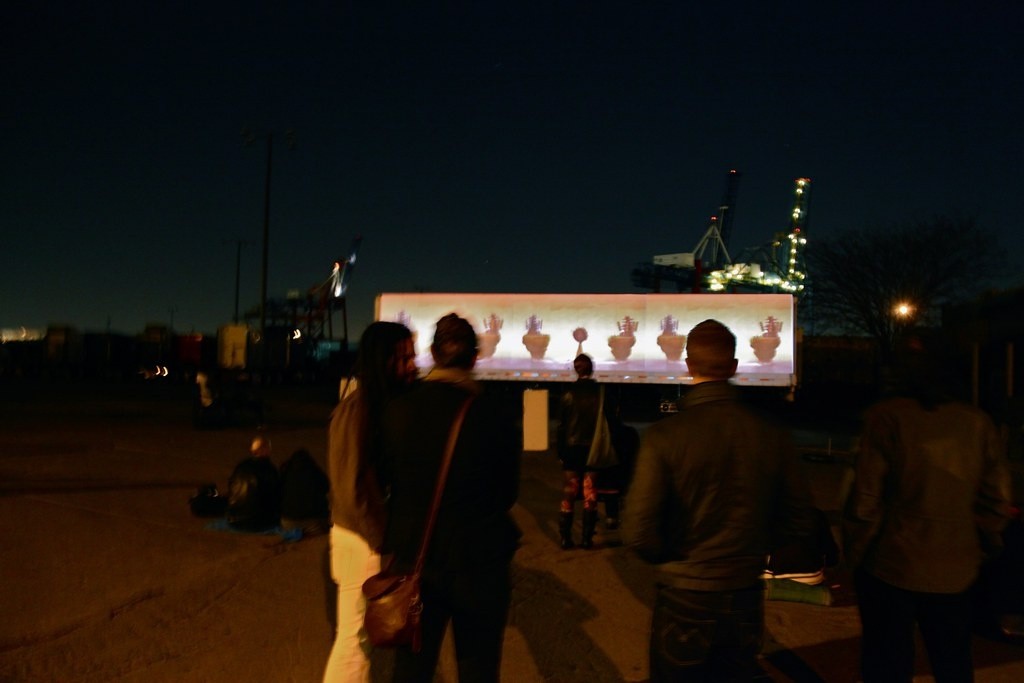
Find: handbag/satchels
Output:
[585,384,618,468]
[361,574,424,654]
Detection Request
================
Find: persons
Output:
[596,399,640,529]
[788,504,841,573]
[226,455,281,532]
[384,311,524,683]
[556,355,616,551]
[321,321,417,683]
[271,448,329,537]
[620,319,814,683]
[838,329,1023,683]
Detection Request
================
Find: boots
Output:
[558,512,575,549]
[582,508,598,548]
